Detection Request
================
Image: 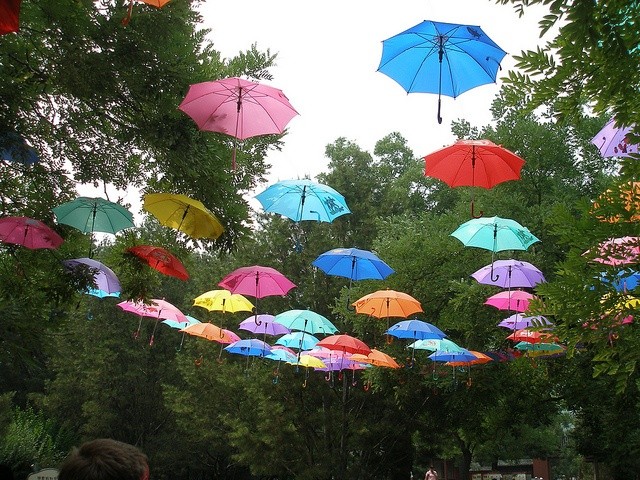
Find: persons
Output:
[424,465,438,480]
[58,439,150,480]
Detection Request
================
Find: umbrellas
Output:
[589,111,640,159]
[385,318,447,369]
[51,197,136,259]
[316,359,345,388]
[359,364,374,368]
[523,348,565,377]
[427,348,479,383]
[581,236,640,294]
[178,77,301,170]
[238,312,292,358]
[223,338,276,377]
[498,312,556,354]
[296,351,327,378]
[448,215,542,282]
[265,350,301,384]
[583,313,634,349]
[310,347,352,381]
[408,337,463,380]
[285,355,328,388]
[214,327,241,364]
[315,332,374,373]
[115,298,164,341]
[274,332,321,373]
[0,0,21,35]
[127,245,190,299]
[351,288,424,344]
[122,0,172,26]
[445,351,492,387]
[350,347,400,391]
[178,320,232,366]
[143,193,226,246]
[252,180,353,253]
[72,284,121,318]
[127,297,189,346]
[505,329,559,368]
[591,290,640,339]
[160,314,201,351]
[192,290,256,339]
[218,265,298,326]
[587,267,640,293]
[272,307,340,345]
[483,288,546,342]
[268,345,297,372]
[513,340,561,368]
[311,247,395,312]
[62,258,123,311]
[570,333,596,352]
[423,140,526,218]
[589,181,640,223]
[375,20,509,124]
[328,349,358,386]
[471,347,521,368]
[536,344,569,360]
[0,217,63,249]
[470,259,548,318]
